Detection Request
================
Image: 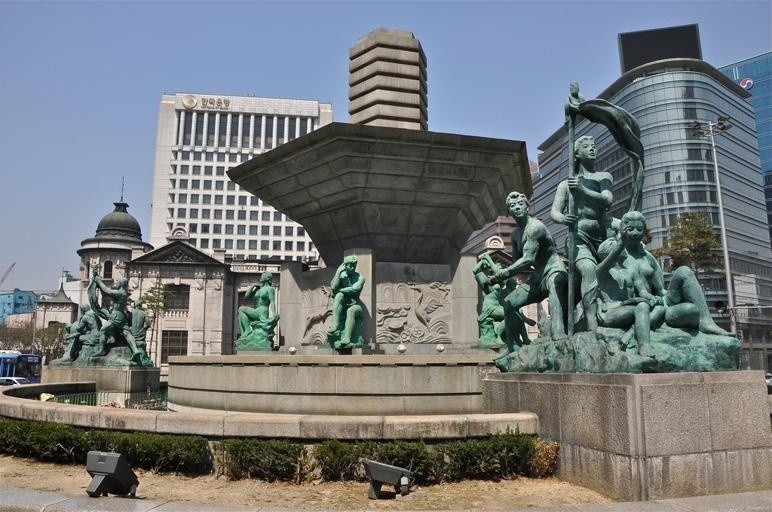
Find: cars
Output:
[0,377,30,385]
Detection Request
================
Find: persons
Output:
[237,271,277,339]
[472,135,735,358]
[58,272,152,362]
[328,255,366,346]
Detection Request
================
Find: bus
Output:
[0,354,41,384]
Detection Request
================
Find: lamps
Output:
[362,457,411,501]
[86,450,141,499]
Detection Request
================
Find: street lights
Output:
[689,116,739,338]
